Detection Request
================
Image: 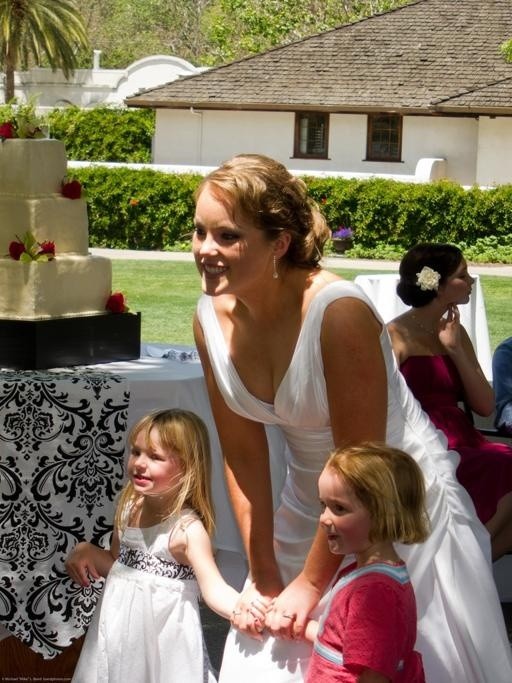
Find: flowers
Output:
[333,227,352,240]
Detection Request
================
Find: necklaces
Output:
[407,309,439,344]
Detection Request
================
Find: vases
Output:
[333,240,353,254]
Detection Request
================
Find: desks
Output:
[1,344,284,683]
[356,272,482,379]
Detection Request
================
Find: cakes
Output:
[0,136,113,316]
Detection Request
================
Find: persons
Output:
[385,240,512,563]
[493,336,512,436]
[188,151,512,682]
[264,439,432,683]
[63,406,263,681]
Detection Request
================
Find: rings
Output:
[232,610,241,618]
[282,612,295,619]
